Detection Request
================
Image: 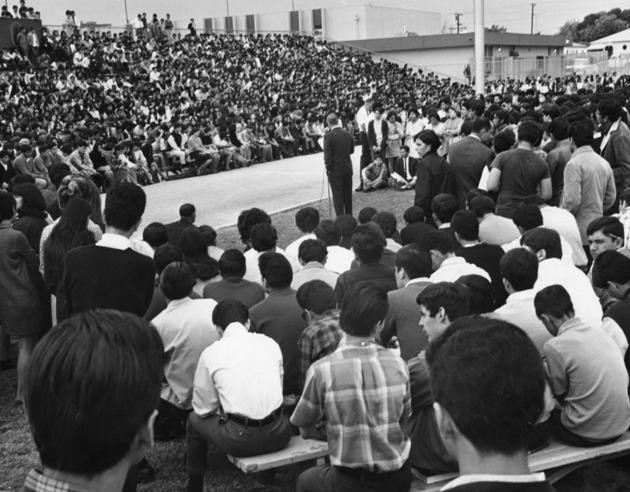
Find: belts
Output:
[341,467,407,480]
[228,407,282,426]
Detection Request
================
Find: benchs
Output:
[411,434,628,492]
[227,436,330,474]
[154,153,180,180]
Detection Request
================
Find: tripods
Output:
[318,166,333,222]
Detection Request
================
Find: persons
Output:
[244,226,295,299]
[486,73,517,149]
[467,195,524,247]
[485,121,551,204]
[413,130,455,207]
[502,199,550,255]
[200,246,264,307]
[356,208,376,224]
[449,118,487,202]
[450,79,486,139]
[236,209,285,258]
[560,118,616,246]
[200,225,227,261]
[46,199,94,301]
[404,208,425,226]
[518,72,572,122]
[453,276,495,318]
[528,195,586,275]
[183,299,290,491]
[369,212,403,254]
[164,204,198,240]
[146,261,221,442]
[464,189,487,208]
[295,278,346,389]
[531,286,630,447]
[325,114,355,215]
[325,37,353,114]
[397,222,432,249]
[174,227,215,279]
[20,304,165,492]
[425,231,488,286]
[336,215,355,247]
[284,207,322,258]
[545,120,574,199]
[573,70,597,119]
[152,244,200,305]
[597,99,629,201]
[582,219,629,276]
[296,283,414,491]
[256,30,324,166]
[488,248,562,452]
[590,249,629,366]
[248,249,312,394]
[1,192,39,411]
[597,69,630,118]
[312,219,353,272]
[431,194,450,229]
[398,281,473,480]
[496,190,521,218]
[520,227,599,328]
[144,223,168,249]
[386,246,450,358]
[39,174,104,271]
[418,67,450,157]
[425,317,555,490]
[610,189,630,250]
[290,236,348,292]
[452,210,513,305]
[1,0,256,191]
[53,180,153,318]
[338,222,401,304]
[11,184,44,248]
[354,44,417,192]
